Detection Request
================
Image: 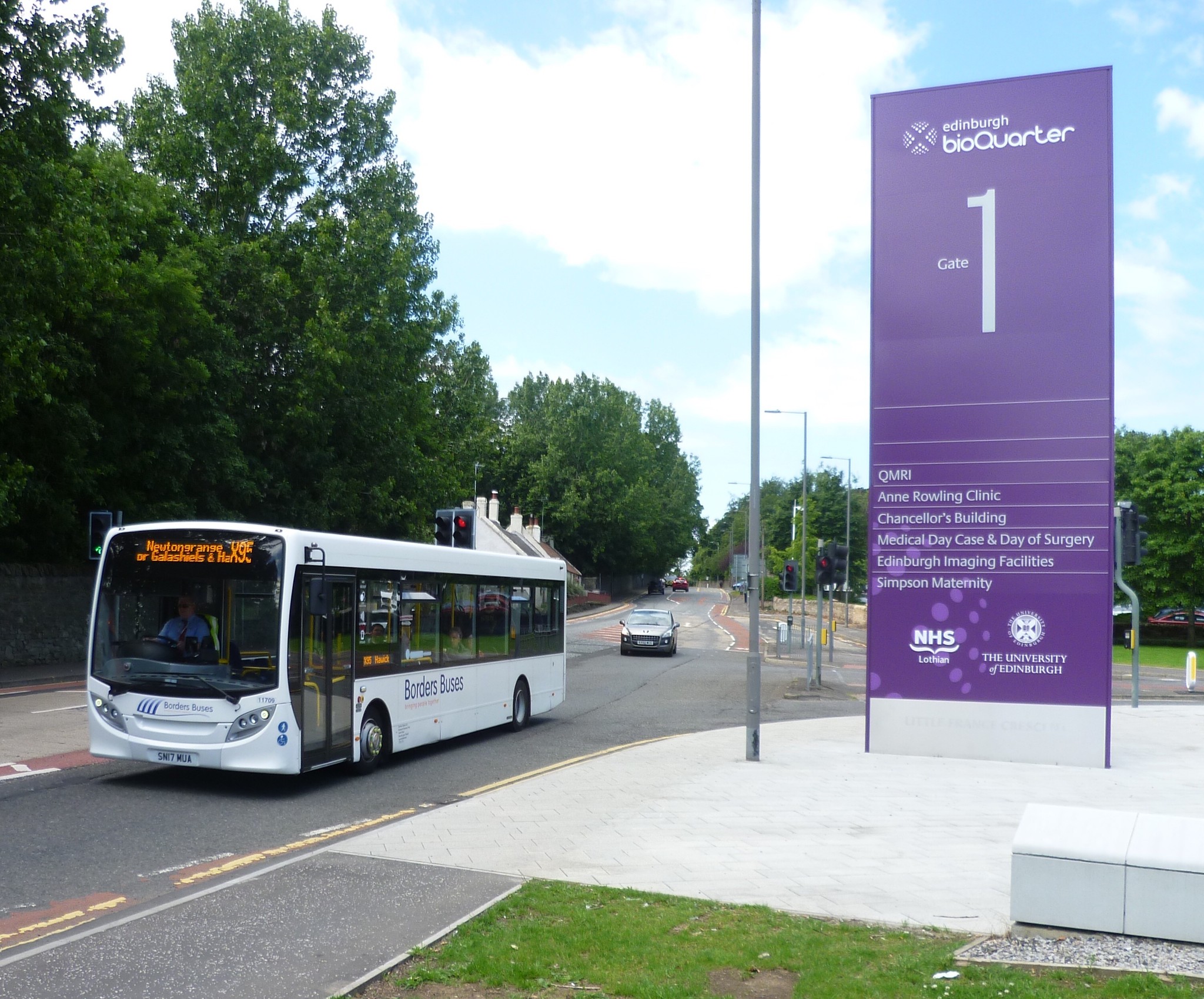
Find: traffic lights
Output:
[452,508,476,550]
[828,541,849,585]
[434,508,454,547]
[1121,511,1149,566]
[815,555,830,585]
[783,560,798,591]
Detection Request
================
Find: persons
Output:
[143,593,210,653]
[368,623,484,658]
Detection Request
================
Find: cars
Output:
[647,580,665,595]
[672,577,689,592]
[1147,605,1204,627]
[732,580,747,591]
[619,608,681,657]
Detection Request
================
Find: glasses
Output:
[178,603,192,608]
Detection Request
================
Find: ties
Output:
[179,621,188,642]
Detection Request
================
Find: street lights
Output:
[723,521,734,566]
[710,541,719,588]
[787,498,805,654]
[734,510,747,559]
[721,530,731,572]
[820,455,852,628]
[764,409,807,648]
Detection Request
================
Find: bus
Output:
[84,521,569,778]
